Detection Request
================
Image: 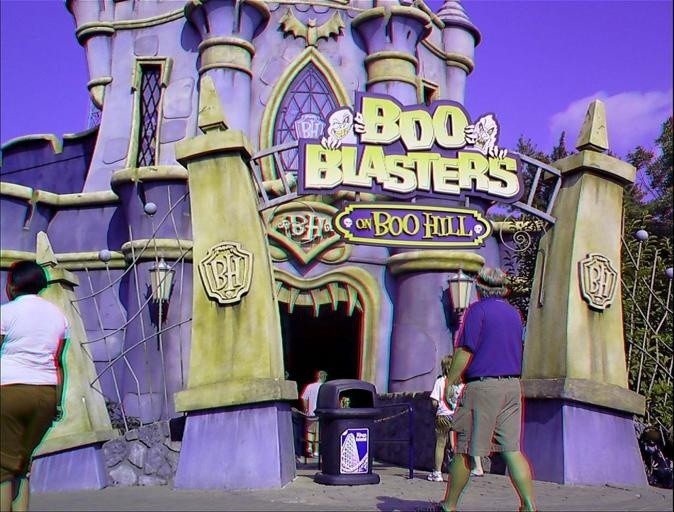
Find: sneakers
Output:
[415,501,447,512]
[307,448,319,458]
[427,470,442,482]
[470,470,483,477]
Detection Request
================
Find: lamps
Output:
[446,265,475,314]
[147,254,176,303]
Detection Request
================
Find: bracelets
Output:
[55,404,60,407]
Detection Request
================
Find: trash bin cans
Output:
[313,379,380,485]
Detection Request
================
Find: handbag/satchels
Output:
[435,416,451,432]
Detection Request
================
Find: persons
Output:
[426,354,464,481]
[0,261,70,511]
[300,370,327,458]
[415,267,539,511]
[468,455,484,477]
